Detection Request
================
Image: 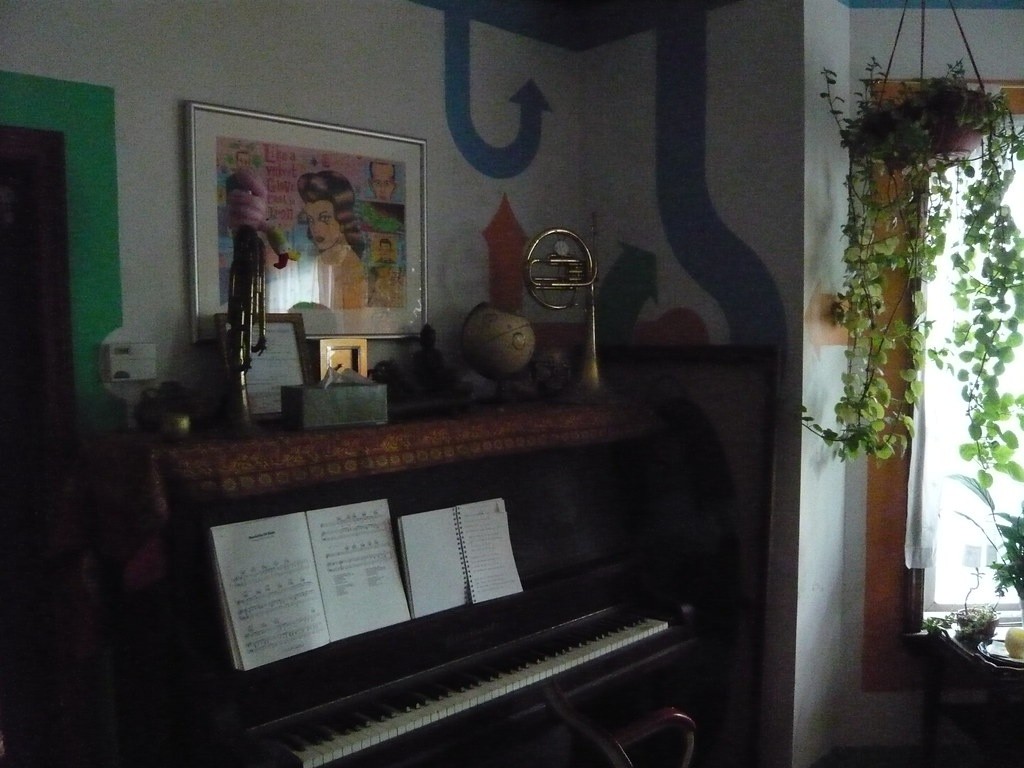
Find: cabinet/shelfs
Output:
[86,395,696,656]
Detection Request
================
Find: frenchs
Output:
[520,211,615,404]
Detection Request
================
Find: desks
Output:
[923,621,1024,768]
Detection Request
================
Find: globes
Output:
[461,303,537,412]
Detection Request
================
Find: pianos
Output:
[89,390,701,768]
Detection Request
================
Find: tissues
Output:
[279,365,391,429]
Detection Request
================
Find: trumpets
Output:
[199,223,270,442]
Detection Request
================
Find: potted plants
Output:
[955,567,1001,643]
[918,618,950,640]
[778,54,1024,489]
[945,474,1024,632]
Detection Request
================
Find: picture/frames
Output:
[184,98,428,342]
[213,311,315,424]
